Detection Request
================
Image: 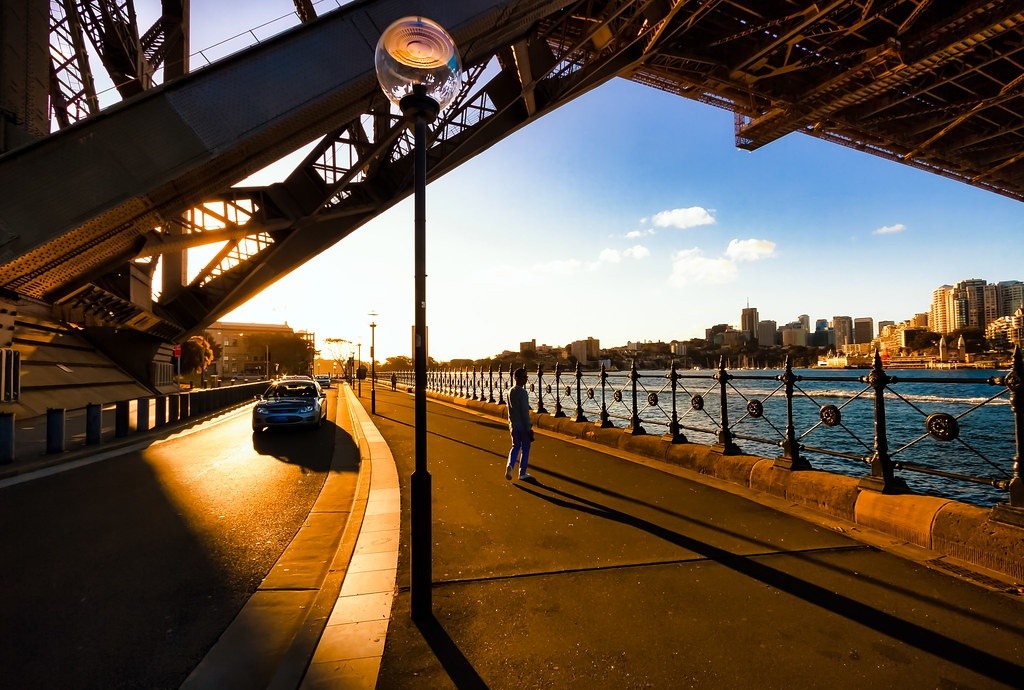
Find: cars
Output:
[276,374,316,382]
[252,379,328,434]
[315,375,332,389]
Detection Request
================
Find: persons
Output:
[505,367,532,480]
[391,373,397,391]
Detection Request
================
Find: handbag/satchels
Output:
[519,424,535,442]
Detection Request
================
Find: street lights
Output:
[371,18,464,621]
[356,341,363,397]
[265,343,271,381]
[317,363,320,375]
[350,349,356,390]
[366,308,380,413]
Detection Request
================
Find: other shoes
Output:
[520,474,531,479]
[506,466,512,480]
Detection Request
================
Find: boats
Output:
[807,348,876,368]
